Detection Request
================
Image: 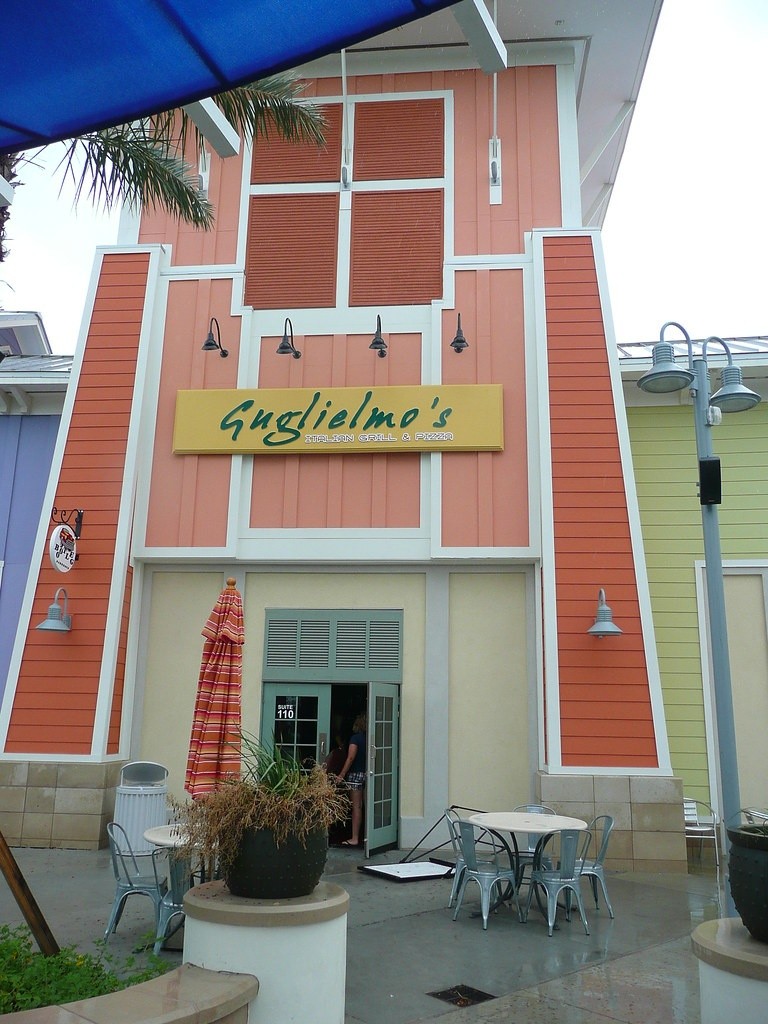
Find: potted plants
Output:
[726,818,768,945]
[164,719,354,900]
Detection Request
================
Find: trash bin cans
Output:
[112,760,169,857]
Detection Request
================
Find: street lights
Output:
[635,321,763,927]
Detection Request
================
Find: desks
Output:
[143,822,220,888]
[469,811,588,930]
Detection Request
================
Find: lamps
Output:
[586,588,623,639]
[34,587,71,634]
[201,317,229,358]
[450,313,469,354]
[369,314,388,359]
[275,318,302,359]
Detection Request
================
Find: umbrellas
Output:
[183,576,245,805]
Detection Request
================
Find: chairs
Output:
[683,797,720,867]
[742,806,768,825]
[102,822,173,945]
[445,808,503,909]
[556,814,614,922]
[508,804,560,906]
[151,845,213,958]
[451,820,524,930]
[522,829,592,937]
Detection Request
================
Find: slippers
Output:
[342,840,359,847]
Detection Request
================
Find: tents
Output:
[0,0,462,154]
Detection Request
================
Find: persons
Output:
[325,728,350,755]
[335,712,367,846]
